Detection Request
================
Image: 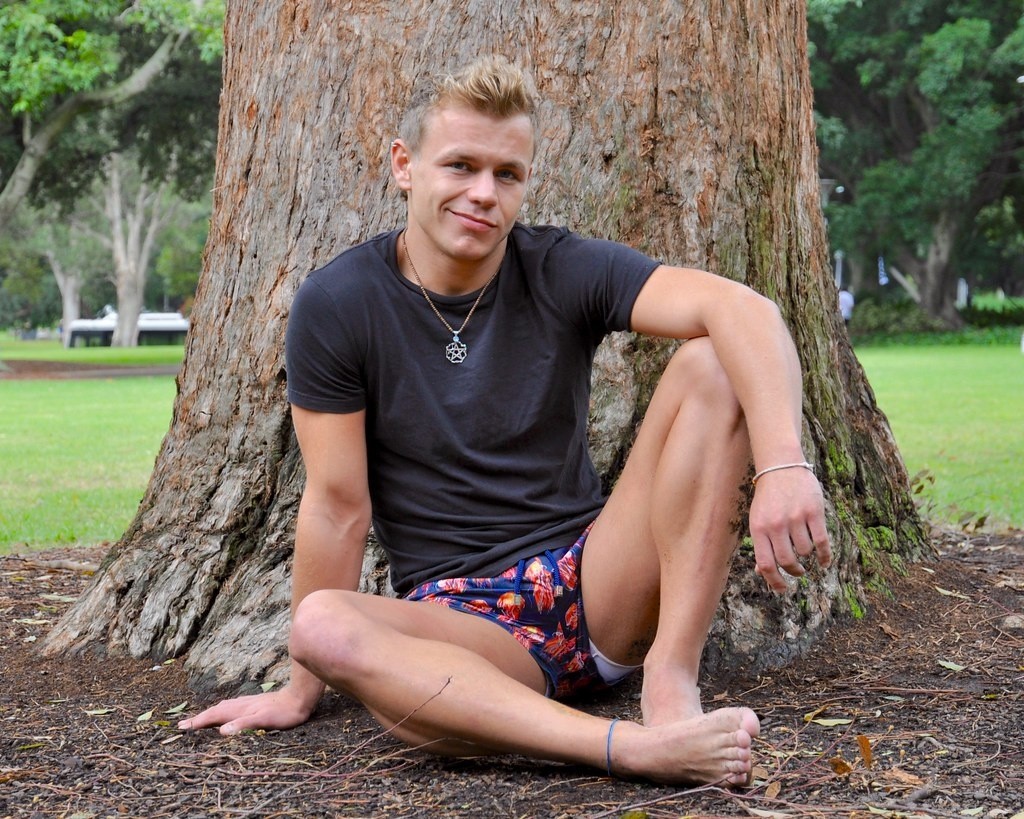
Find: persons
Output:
[839,287,854,328]
[177,57,829,789]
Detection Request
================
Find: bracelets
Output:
[751,462,814,485]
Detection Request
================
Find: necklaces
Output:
[402,227,506,364]
